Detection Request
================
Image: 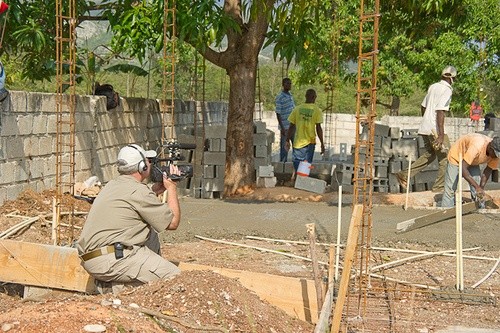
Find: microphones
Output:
[178,143,197,149]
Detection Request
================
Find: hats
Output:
[117,143,157,175]
[491,136,500,158]
[441,65,457,78]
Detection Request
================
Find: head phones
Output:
[125,144,148,175]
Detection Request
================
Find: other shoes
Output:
[395,170,408,190]
[85,275,99,295]
[431,186,445,192]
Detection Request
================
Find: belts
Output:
[79,244,126,262]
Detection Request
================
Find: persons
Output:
[285,88,326,180]
[441,133,499,207]
[398,63,458,192]
[470,100,482,124]
[275,78,297,162]
[77,144,181,296]
